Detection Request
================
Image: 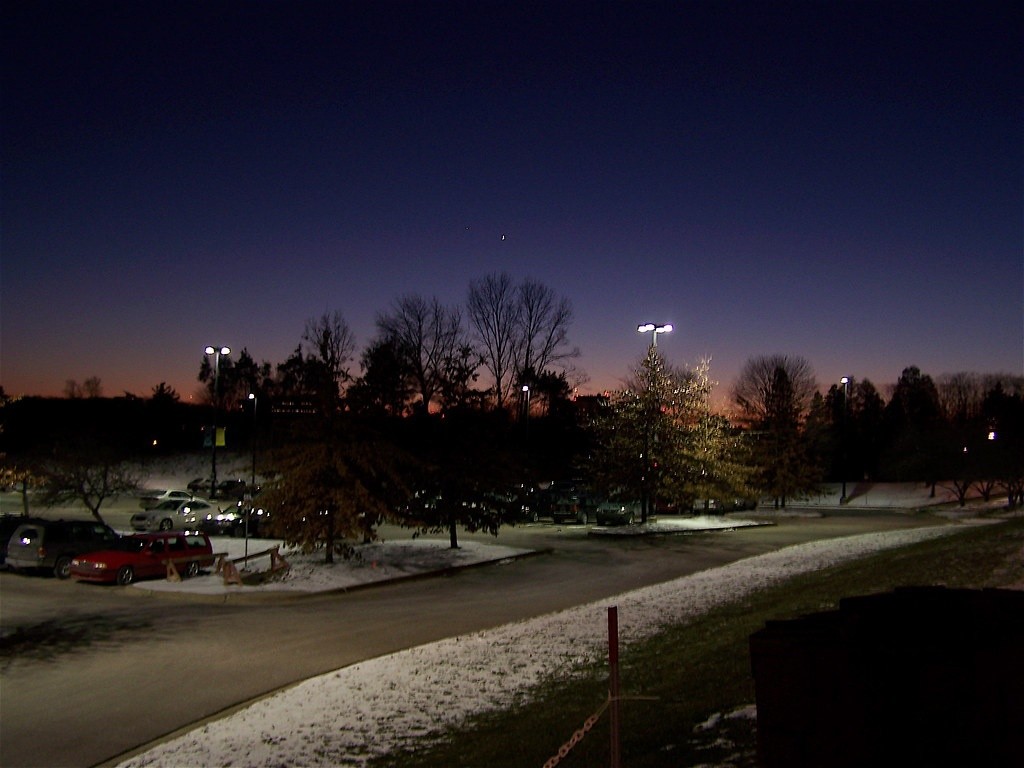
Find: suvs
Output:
[0,515,122,580]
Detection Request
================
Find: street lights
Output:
[249,393,258,498]
[522,385,530,432]
[840,376,849,504]
[204,345,232,500]
[637,322,674,524]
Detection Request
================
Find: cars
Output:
[595,492,642,526]
[502,497,542,524]
[129,477,273,539]
[69,531,217,587]
[552,496,596,525]
